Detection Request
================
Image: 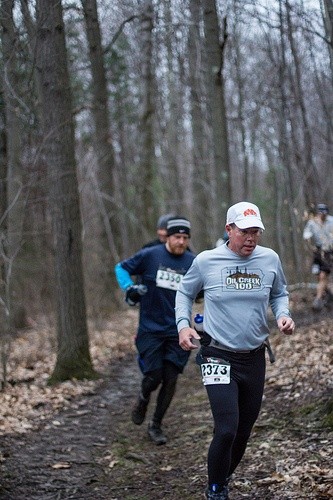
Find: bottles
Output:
[194,314,204,332]
[126,284,148,306]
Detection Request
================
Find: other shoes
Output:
[311,299,322,311]
[322,287,332,300]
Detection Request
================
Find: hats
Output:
[157,215,173,230]
[166,217,192,238]
[317,203,331,215]
[226,201,265,231]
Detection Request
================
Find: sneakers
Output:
[205,484,229,500]
[131,393,149,425]
[148,422,167,447]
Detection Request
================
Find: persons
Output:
[115,216,204,445]
[133,204,333,313]
[175,202,296,500]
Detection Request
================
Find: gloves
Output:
[127,286,142,303]
